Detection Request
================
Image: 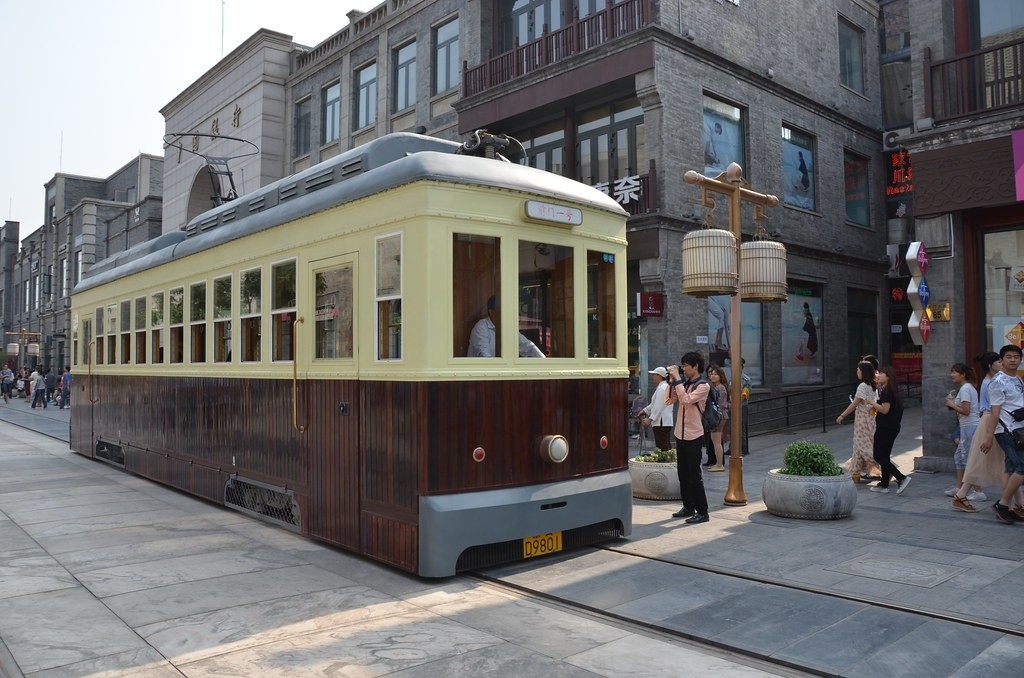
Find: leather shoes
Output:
[672,507,695,517]
[685,513,709,523]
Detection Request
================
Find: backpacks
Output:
[691,379,723,433]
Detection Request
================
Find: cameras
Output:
[666,366,671,373]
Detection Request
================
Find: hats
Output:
[647,367,668,379]
[741,357,745,365]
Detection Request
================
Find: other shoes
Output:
[707,464,724,472]
[869,485,890,494]
[860,474,882,482]
[15,394,20,398]
[703,461,712,466]
[63,405,70,409]
[896,475,911,494]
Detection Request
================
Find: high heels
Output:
[851,472,860,483]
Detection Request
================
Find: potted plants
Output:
[629,446,684,501]
[762,440,858,520]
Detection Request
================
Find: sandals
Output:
[1012,502,1024,517]
[951,495,980,512]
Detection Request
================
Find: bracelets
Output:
[841,415,844,418]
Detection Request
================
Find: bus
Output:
[69,126,632,582]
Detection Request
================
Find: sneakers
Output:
[944,487,960,497]
[993,509,1024,525]
[965,490,987,501]
[992,499,1014,524]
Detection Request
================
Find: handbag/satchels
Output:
[1011,426,1024,450]
[1010,408,1024,422]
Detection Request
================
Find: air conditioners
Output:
[882,128,911,153]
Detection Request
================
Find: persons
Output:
[947,344,1024,522]
[702,117,723,166]
[0,364,71,409]
[836,355,911,494]
[469,295,547,359]
[663,351,710,524]
[793,151,809,192]
[638,357,750,472]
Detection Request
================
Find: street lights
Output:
[5,328,42,395]
[681,162,789,506]
